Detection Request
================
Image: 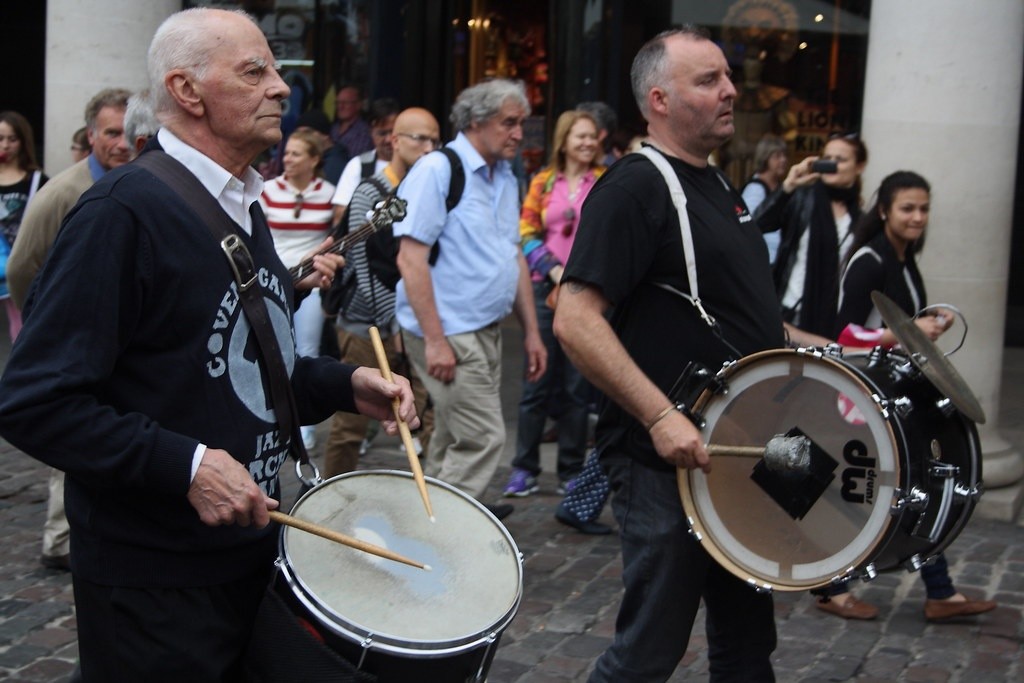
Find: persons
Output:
[0,76,998,626]
[552,25,880,683]
[0,9,424,683]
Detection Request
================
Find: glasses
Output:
[396,133,442,150]
[334,99,362,105]
[294,193,304,219]
[71,146,81,150]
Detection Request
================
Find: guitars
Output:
[288,196,409,286]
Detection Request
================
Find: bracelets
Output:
[645,404,674,432]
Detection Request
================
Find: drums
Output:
[675,344,986,594]
[271,465,526,682]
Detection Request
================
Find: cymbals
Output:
[870,289,987,424]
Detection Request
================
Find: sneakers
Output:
[555,480,571,495]
[502,467,541,497]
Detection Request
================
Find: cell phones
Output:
[812,161,837,173]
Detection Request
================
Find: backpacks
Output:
[365,147,465,292]
[319,178,390,315]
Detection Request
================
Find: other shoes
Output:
[555,506,611,535]
[482,504,514,520]
[39,553,72,569]
[358,439,371,455]
[399,437,424,456]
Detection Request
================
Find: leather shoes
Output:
[816,592,880,620]
[923,592,998,620]
